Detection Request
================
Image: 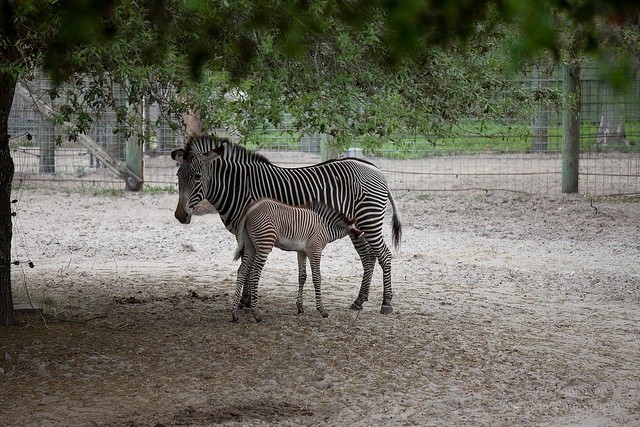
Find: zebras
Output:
[230,196,360,322]
[171,133,404,314]
